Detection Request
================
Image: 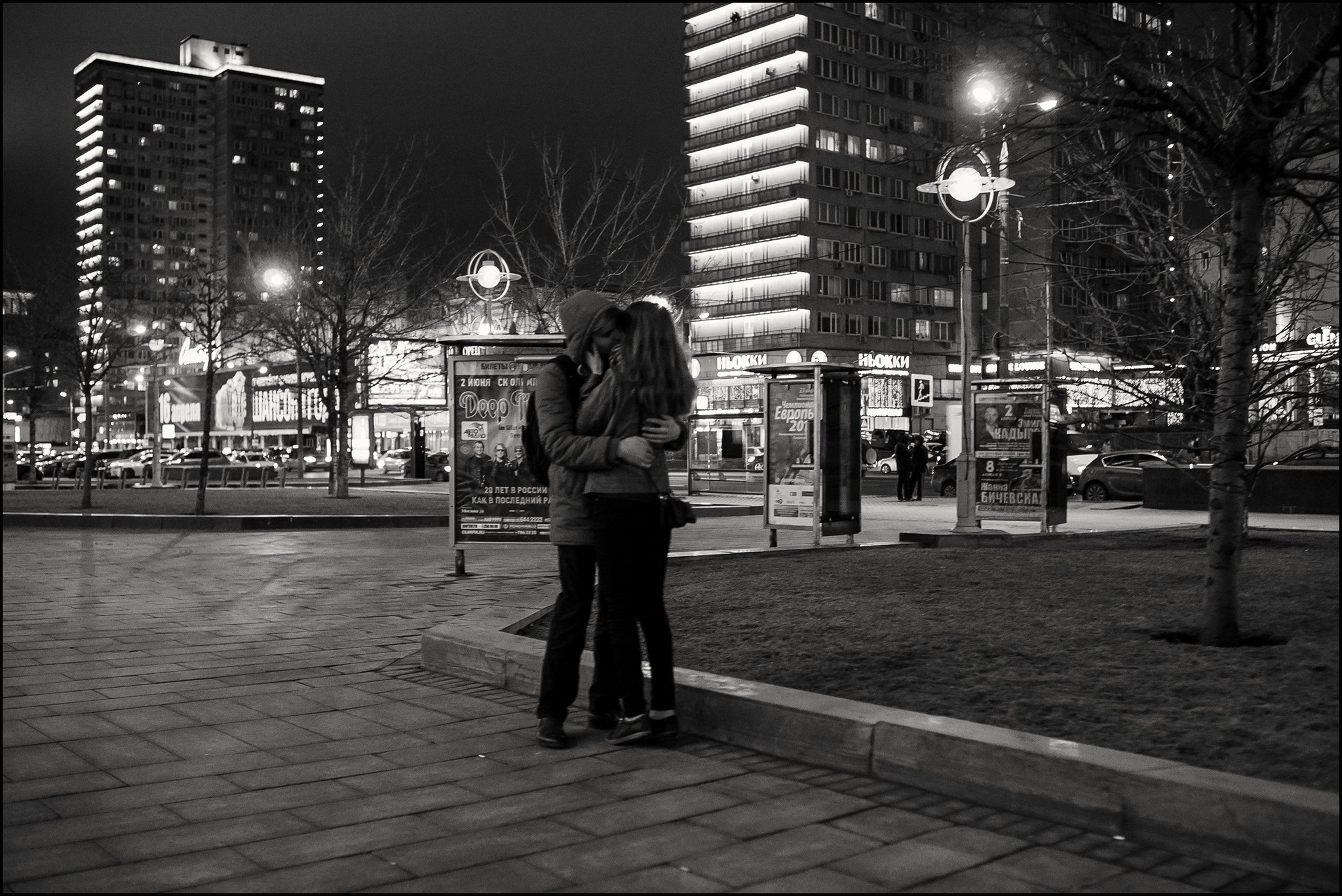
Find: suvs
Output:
[861,438,878,476]
[744,447,765,459]
[1080,448,1186,502]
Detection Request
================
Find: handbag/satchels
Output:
[662,497,696,529]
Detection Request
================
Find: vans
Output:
[1067,429,1100,491]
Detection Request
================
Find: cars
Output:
[877,442,948,475]
[931,452,956,498]
[1273,445,1339,467]
[374,447,449,481]
[17,447,289,479]
[744,454,764,470]
[284,448,331,471]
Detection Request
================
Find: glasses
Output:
[496,449,505,452]
[514,450,522,452]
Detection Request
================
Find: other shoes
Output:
[898,497,904,501]
[535,719,574,747]
[906,498,913,501]
[916,497,922,501]
[588,711,622,731]
[649,716,678,733]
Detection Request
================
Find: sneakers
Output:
[606,715,651,745]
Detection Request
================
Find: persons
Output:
[871,431,904,450]
[465,441,537,513]
[896,434,928,502]
[976,405,1010,451]
[1188,435,1200,461]
[534,289,688,749]
[732,11,740,18]
[1102,438,1111,454]
[574,302,696,745]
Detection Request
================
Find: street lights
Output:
[264,268,302,478]
[456,249,523,334]
[916,143,1015,532]
[61,387,74,450]
[967,71,1060,377]
[142,328,176,486]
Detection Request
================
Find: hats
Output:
[915,435,925,443]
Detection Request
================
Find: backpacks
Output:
[521,354,579,487]
